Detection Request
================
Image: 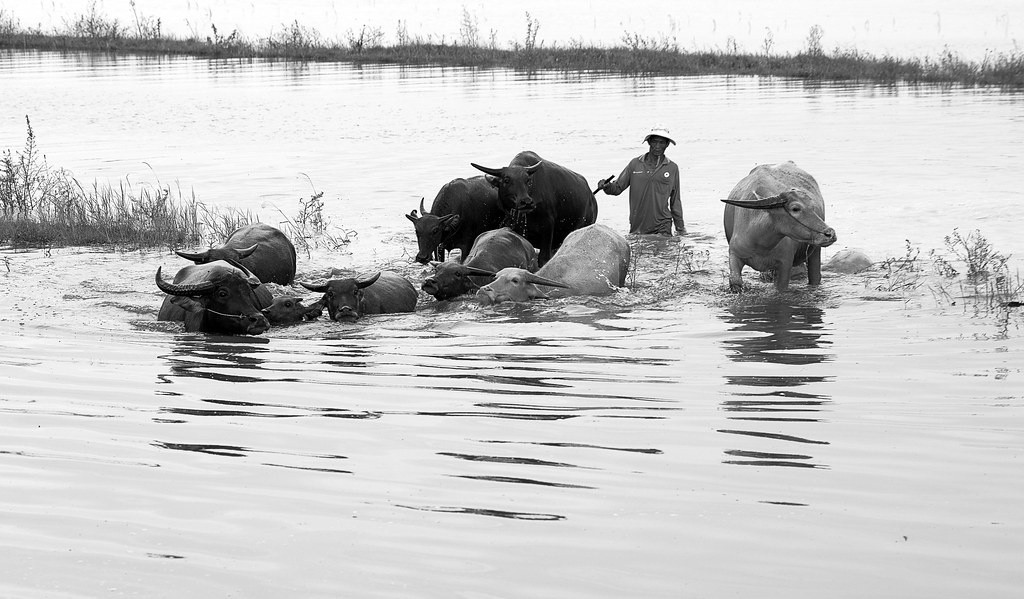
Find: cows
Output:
[155,150,631,336]
[719,159,875,295]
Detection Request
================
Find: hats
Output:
[642,125,676,145]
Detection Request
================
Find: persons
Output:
[598,128,684,233]
